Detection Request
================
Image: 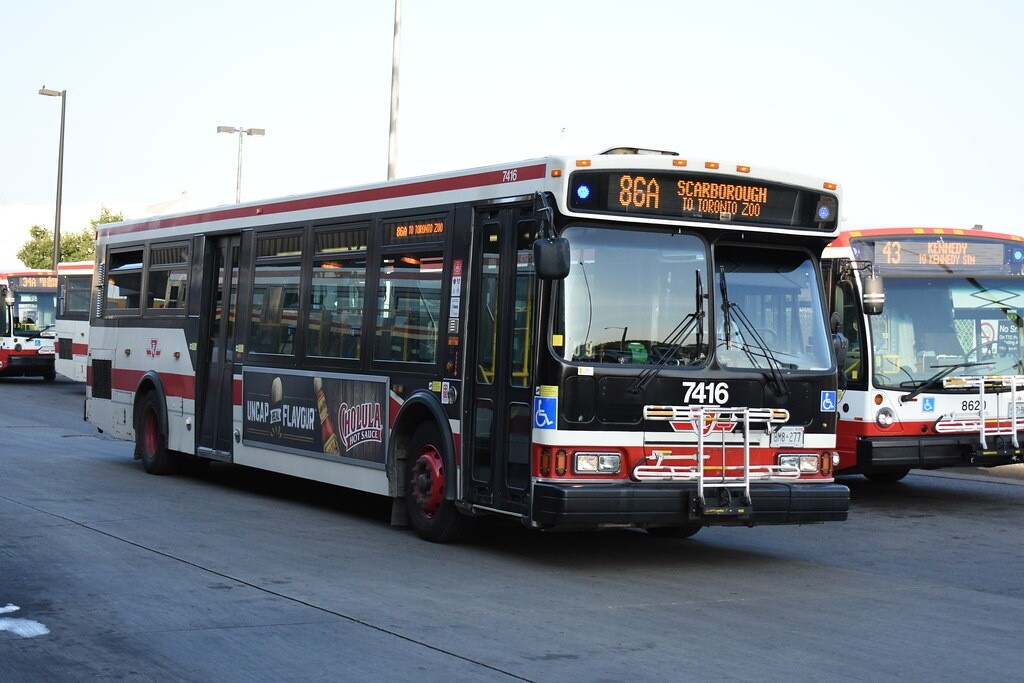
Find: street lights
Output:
[38,86,67,269]
[217,125,267,203]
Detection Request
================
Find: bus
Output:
[0,222,1024,486]
[84,134,848,544]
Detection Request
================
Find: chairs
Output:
[163,281,186,310]
